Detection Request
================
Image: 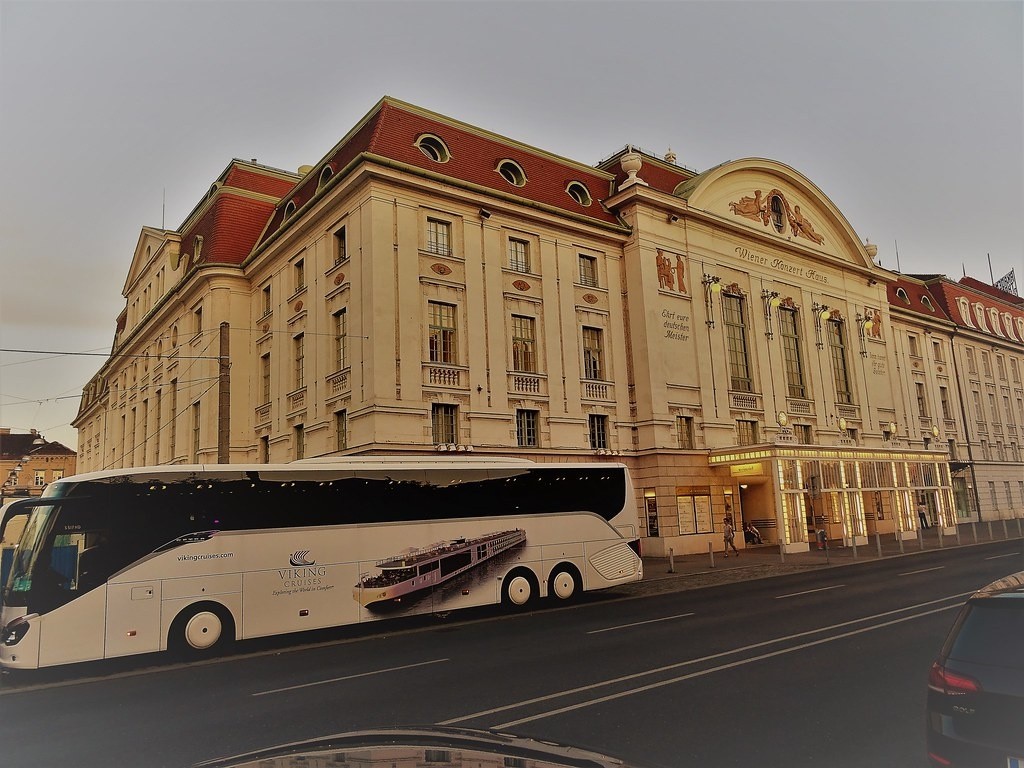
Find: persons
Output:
[917,502,931,529]
[746,523,764,545]
[723,518,740,558]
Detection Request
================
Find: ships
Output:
[351,528,526,611]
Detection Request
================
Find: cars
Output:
[928,572,1024,768]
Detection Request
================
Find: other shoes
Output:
[724,555,728,557]
[758,542,764,544]
[736,551,739,556]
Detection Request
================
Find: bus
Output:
[0,455,644,678]
